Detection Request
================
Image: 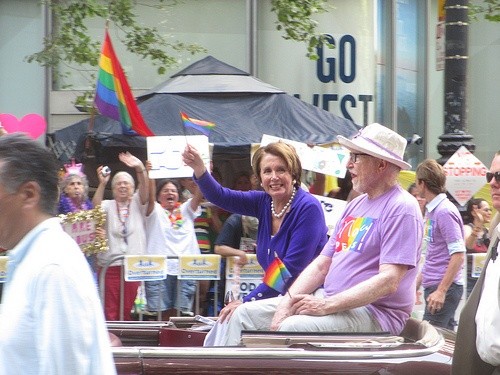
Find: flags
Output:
[178,111,217,136]
[91,29,155,136]
[261,257,292,295]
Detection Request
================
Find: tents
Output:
[50,54,364,161]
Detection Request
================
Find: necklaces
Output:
[159,201,182,230]
[114,198,132,243]
[271,187,296,218]
[65,198,88,214]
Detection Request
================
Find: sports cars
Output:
[101,316,458,375]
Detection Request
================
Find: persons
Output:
[183,144,328,323]
[0,132,117,374]
[221,123,425,346]
[57,152,500,374]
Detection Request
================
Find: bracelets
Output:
[470,233,480,238]
[473,226,482,229]
[473,229,481,233]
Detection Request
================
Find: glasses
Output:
[479,208,492,212]
[349,152,368,162]
[486,171,500,183]
[160,189,179,195]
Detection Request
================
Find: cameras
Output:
[101,166,111,176]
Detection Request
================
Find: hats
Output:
[336,122,412,171]
[62,158,87,182]
